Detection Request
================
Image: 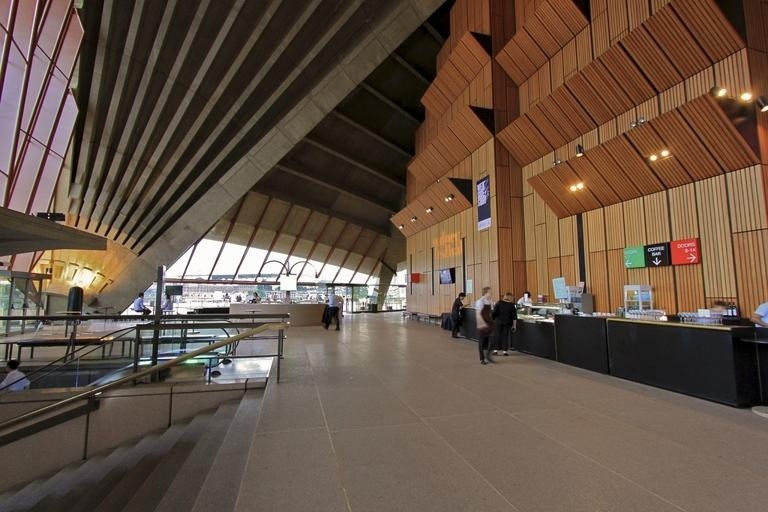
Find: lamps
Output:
[426,207,433,214]
[756,96,768,113]
[411,216,417,222]
[575,143,583,158]
[445,194,454,202]
[710,86,726,97]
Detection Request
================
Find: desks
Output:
[162,310,172,336]
[243,310,262,335]
[96,306,113,331]
[56,311,81,337]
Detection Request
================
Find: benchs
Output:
[402,311,418,320]
[416,313,440,325]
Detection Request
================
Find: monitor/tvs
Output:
[440,268,455,284]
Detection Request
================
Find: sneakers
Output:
[492,352,509,356]
[480,358,493,365]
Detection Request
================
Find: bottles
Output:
[615,305,666,321]
[627,292,649,305]
[713,301,742,321]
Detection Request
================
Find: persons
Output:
[321,292,340,331]
[451,292,470,338]
[134,292,151,320]
[475,287,494,364]
[750,301,768,356]
[517,292,533,306]
[252,292,260,303]
[161,295,173,313]
[0,360,30,393]
[492,292,517,355]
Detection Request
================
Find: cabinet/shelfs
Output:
[560,294,594,314]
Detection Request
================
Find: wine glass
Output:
[677,310,724,327]
